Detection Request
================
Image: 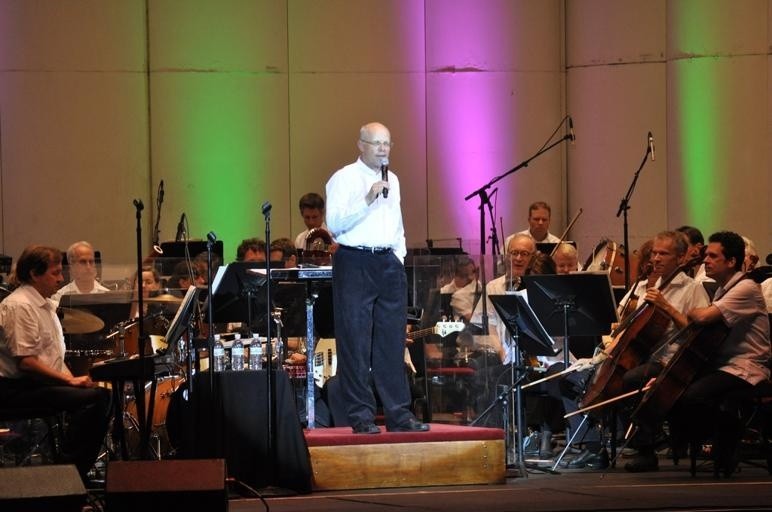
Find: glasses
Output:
[509,249,536,257]
[360,139,394,149]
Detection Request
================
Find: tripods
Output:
[506,306,594,478]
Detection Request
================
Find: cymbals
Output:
[55,305,105,335]
[130,293,186,304]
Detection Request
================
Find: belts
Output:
[339,244,393,256]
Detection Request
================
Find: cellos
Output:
[628,255,771,427]
[576,243,706,426]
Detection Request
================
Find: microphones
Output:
[381,158,388,198]
[648,131,657,161]
[176,212,185,243]
[568,117,577,145]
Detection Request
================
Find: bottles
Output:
[214,333,283,371]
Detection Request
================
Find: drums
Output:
[113,316,169,359]
[582,239,639,286]
[121,373,184,455]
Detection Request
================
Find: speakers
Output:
[104,459,230,511]
[0,464,88,512]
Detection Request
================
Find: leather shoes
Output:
[623,454,659,473]
[553,447,610,471]
[352,422,381,434]
[539,429,553,459]
[385,416,430,433]
[78,473,108,490]
[622,432,672,457]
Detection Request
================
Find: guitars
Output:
[313,314,465,389]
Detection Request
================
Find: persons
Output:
[324,120,430,435]
